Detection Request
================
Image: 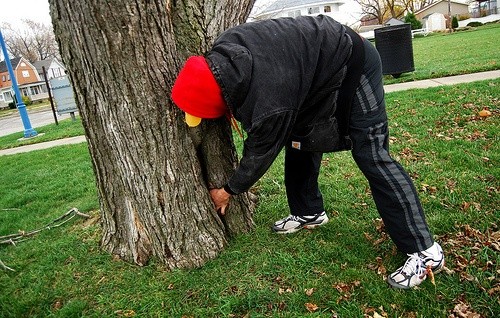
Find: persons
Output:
[170,12,446,289]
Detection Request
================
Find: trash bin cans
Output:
[372,22,416,79]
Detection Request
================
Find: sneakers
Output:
[273,210,329,234]
[387,241,446,289]
